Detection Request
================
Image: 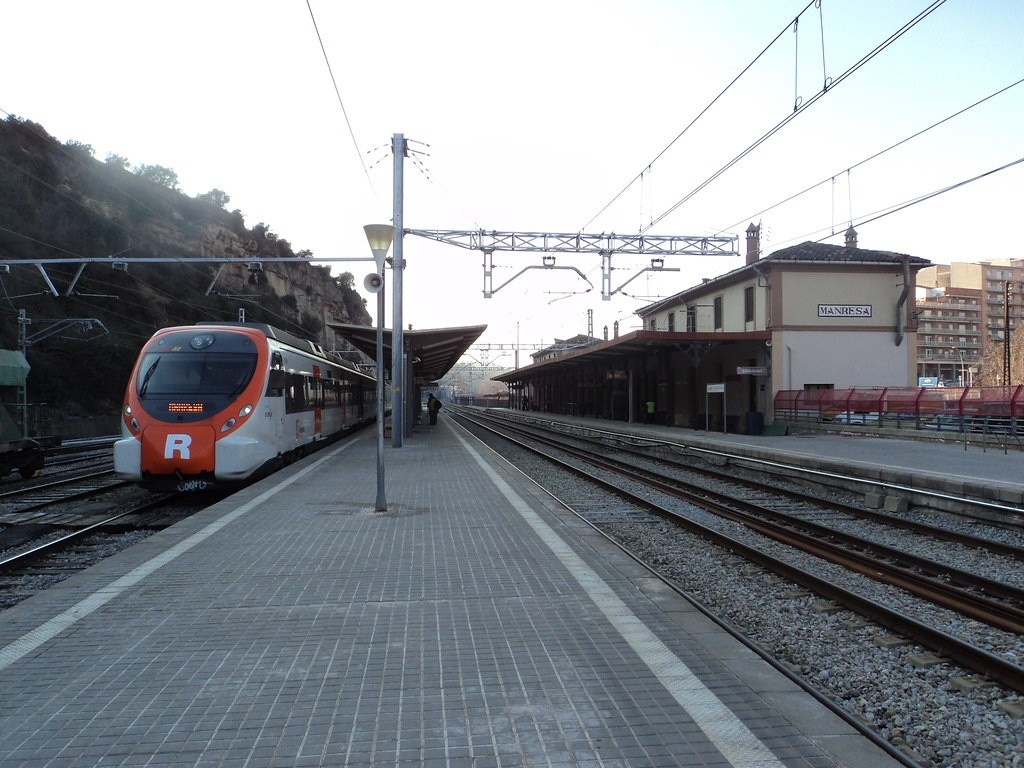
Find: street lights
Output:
[951,346,964,387]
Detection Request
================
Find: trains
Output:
[113,321,391,486]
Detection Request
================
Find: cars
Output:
[819,403,1024,435]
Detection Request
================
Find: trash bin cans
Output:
[384,408,392,438]
[745,410,764,436]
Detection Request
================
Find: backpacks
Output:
[434,400,441,410]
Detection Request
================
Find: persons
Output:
[642,396,657,425]
[522,395,528,411]
[427,393,439,425]
